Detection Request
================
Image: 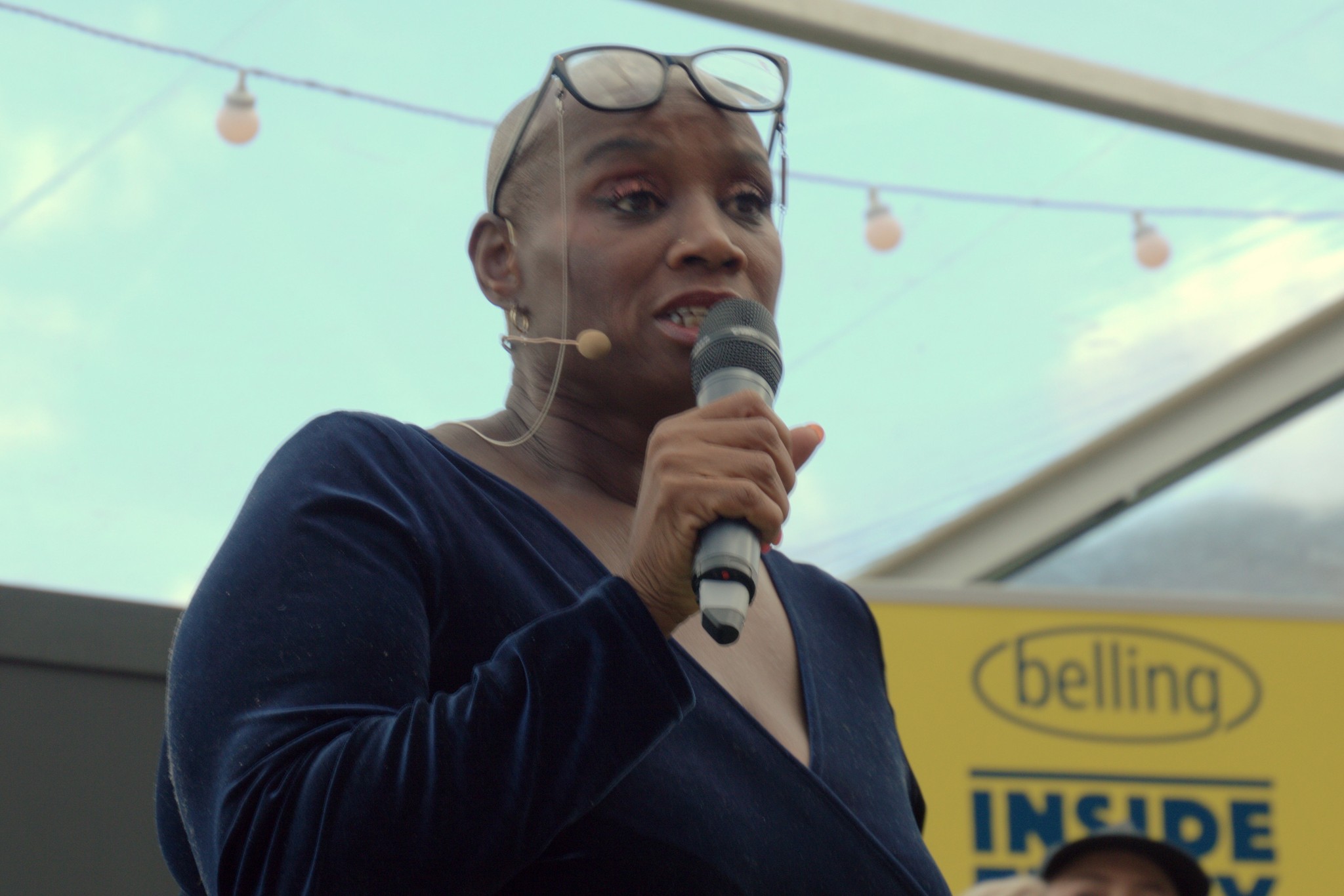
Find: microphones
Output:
[690,297,784,644]
[501,329,613,361]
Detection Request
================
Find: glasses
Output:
[493,45,788,217]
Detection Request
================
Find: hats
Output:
[1041,824,1210,896]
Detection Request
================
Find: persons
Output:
[1042,826,1210,896]
[157,59,969,896]
